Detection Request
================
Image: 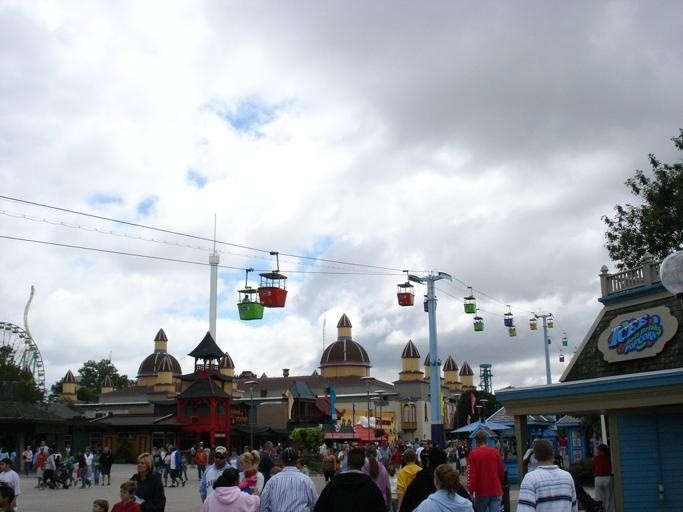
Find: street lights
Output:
[359,376,377,446]
[452,393,461,428]
[374,388,384,446]
[369,394,380,434]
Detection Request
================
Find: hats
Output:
[214,446,227,456]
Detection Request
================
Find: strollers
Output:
[45,462,70,488]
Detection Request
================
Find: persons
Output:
[190,440,319,512]
[241,295,250,304]
[92,452,166,512]
[22,441,111,490]
[516,432,612,512]
[313,429,504,512]
[151,443,187,488]
[1,446,21,512]
